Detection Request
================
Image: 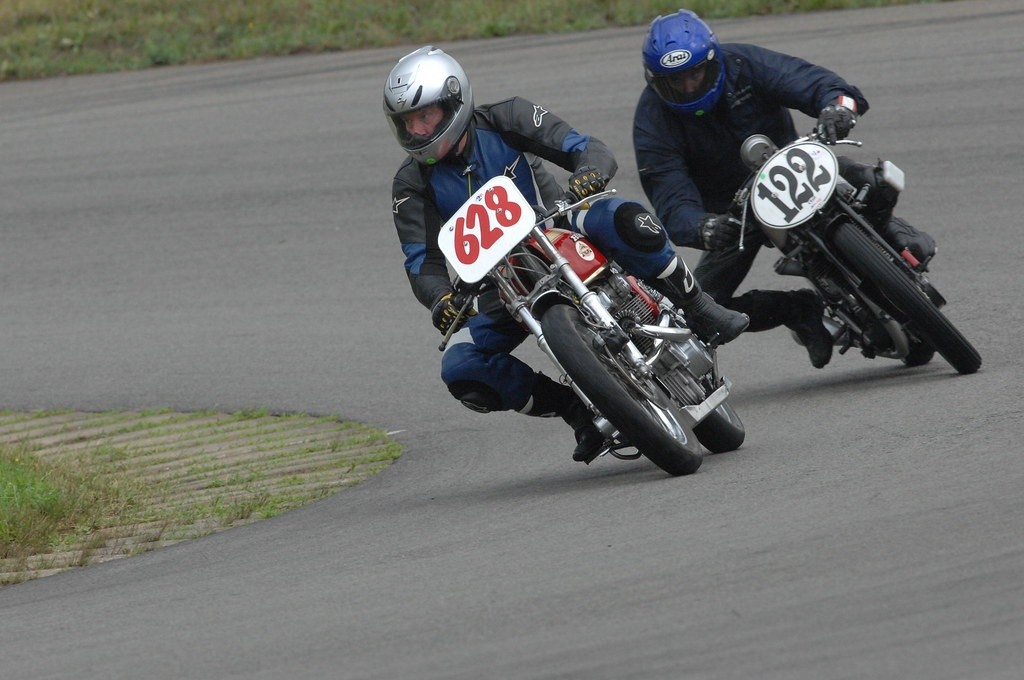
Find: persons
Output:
[381,42,750,460]
[632,6,936,370]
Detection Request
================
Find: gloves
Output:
[697,213,748,250]
[815,96,858,145]
[428,286,478,336]
[567,165,606,209]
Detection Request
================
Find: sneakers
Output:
[882,215,936,260]
[561,394,604,461]
[788,288,833,369]
[678,290,750,344]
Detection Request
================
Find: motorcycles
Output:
[431,165,746,478]
[716,112,982,377]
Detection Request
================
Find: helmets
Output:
[382,45,475,165]
[642,9,727,117]
